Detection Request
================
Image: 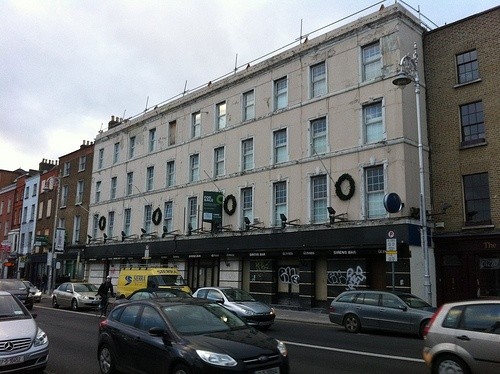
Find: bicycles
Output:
[96,297,113,319]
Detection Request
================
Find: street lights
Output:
[42,175,60,295]
[391,42,432,306]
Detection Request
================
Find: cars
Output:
[23,281,42,303]
[420,300,500,374]
[97,299,290,374]
[193,286,276,329]
[0,279,33,310]
[50,282,102,311]
[112,288,200,309]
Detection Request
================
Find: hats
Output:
[107,276,111,278]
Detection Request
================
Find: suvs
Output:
[0,291,50,374]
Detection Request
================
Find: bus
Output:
[329,291,464,335]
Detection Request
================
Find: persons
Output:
[54,272,71,286]
[95,276,116,311]
[41,271,47,294]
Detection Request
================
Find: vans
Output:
[117,266,191,299]
